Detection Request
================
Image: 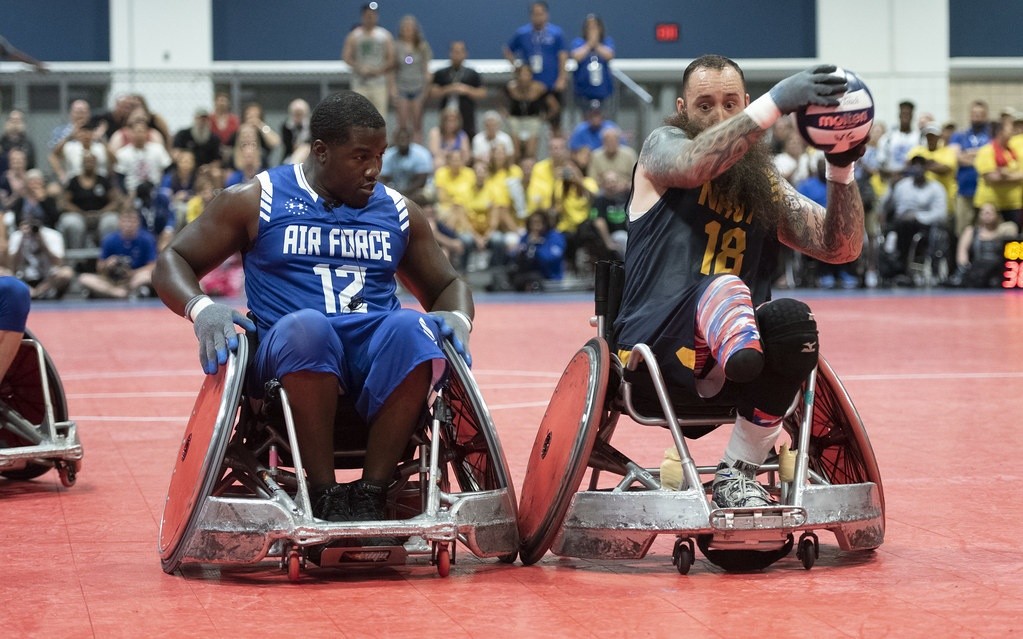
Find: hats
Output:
[924,121,942,136]
[194,107,208,116]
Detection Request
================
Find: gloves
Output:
[825,136,871,185]
[427,311,472,370]
[186,295,257,375]
[744,63,849,131]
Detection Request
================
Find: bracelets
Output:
[452,309,473,334]
[189,295,215,323]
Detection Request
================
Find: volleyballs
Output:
[795,66,876,155]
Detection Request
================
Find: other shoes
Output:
[818,269,859,290]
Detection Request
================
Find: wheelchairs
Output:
[0,322,79,492]
[159,328,517,577]
[519,335,885,573]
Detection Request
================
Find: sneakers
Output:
[712,459,781,513]
[308,480,403,564]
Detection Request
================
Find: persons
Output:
[617,49,867,512]
[0,0,1022,304]
[0,271,30,382]
[152,93,473,523]
